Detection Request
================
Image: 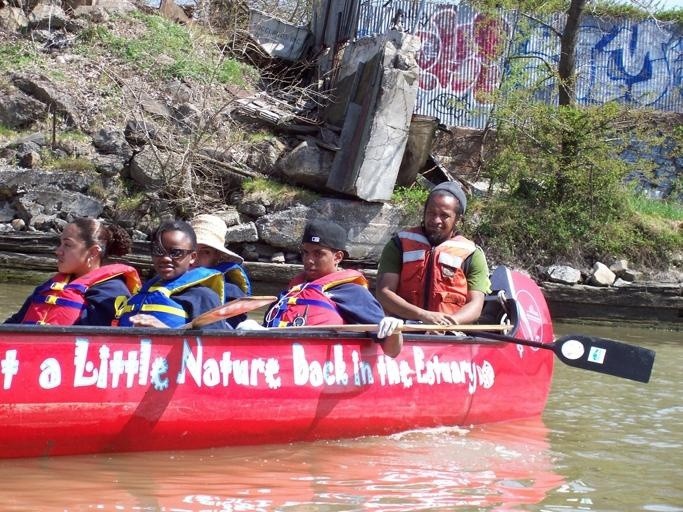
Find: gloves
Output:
[377,317,403,339]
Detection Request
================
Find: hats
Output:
[302,221,349,258]
[190,214,244,264]
[430,182,467,214]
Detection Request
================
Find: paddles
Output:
[461,330,655,382]
[176,295,278,328]
[266,324,512,332]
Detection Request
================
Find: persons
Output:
[1,216,135,326]
[374,180,493,337]
[179,212,254,333]
[100,219,228,331]
[257,219,404,360]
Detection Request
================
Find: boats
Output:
[0,263,556,461]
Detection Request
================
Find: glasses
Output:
[151,247,194,259]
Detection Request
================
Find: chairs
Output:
[475,297,519,336]
